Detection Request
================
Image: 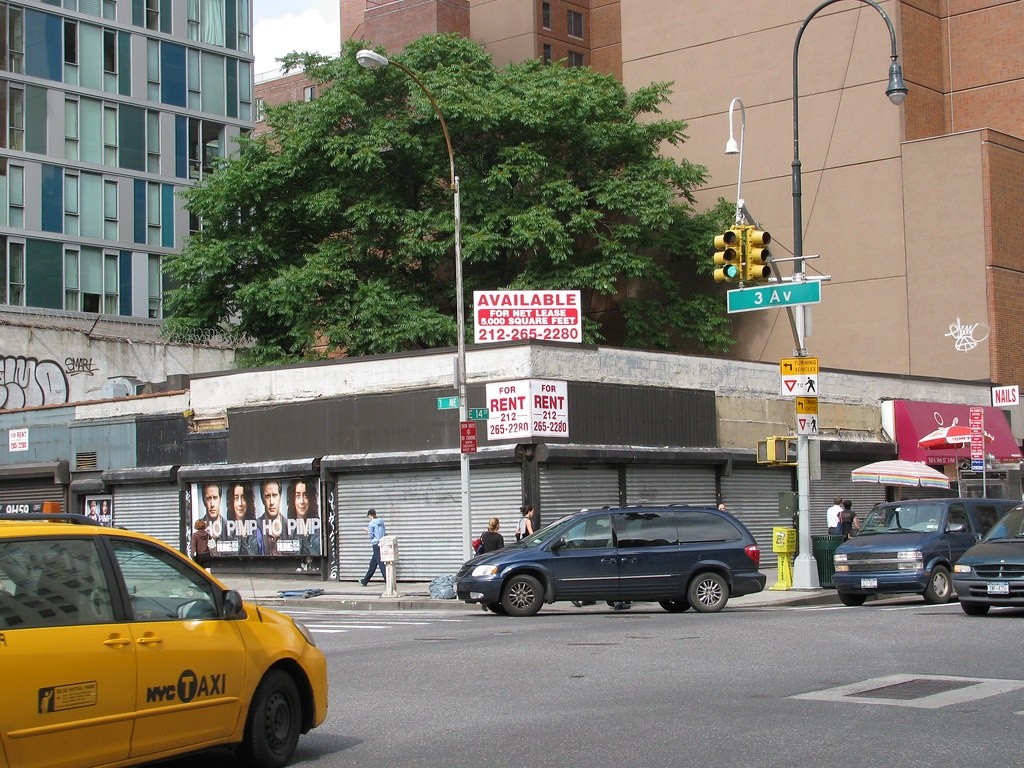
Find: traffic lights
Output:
[757,440,773,463]
[722,229,743,282]
[713,234,727,283]
[745,229,772,282]
[766,440,788,462]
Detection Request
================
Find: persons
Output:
[191,519,211,575]
[100,500,110,522]
[257,479,288,556]
[358,508,387,586]
[480,517,504,612]
[717,502,726,511]
[826,497,860,539]
[515,504,535,542]
[200,483,227,556]
[286,479,320,555]
[226,481,258,555]
[88,500,98,522]
[571,506,631,609]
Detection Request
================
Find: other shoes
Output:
[571,600,632,609]
[359,577,364,586]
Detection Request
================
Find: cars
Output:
[952,502,1024,616]
[0,511,331,768]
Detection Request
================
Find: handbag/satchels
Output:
[207,535,216,549]
[472,533,484,552]
[835,512,843,535]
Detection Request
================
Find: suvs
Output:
[450,504,769,619]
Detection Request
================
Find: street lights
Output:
[787,0,910,592]
[353,48,472,569]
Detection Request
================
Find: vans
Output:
[830,495,1024,606]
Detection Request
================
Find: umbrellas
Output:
[850,459,950,500]
[917,425,993,498]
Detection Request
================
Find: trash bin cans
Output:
[810,534,847,589]
